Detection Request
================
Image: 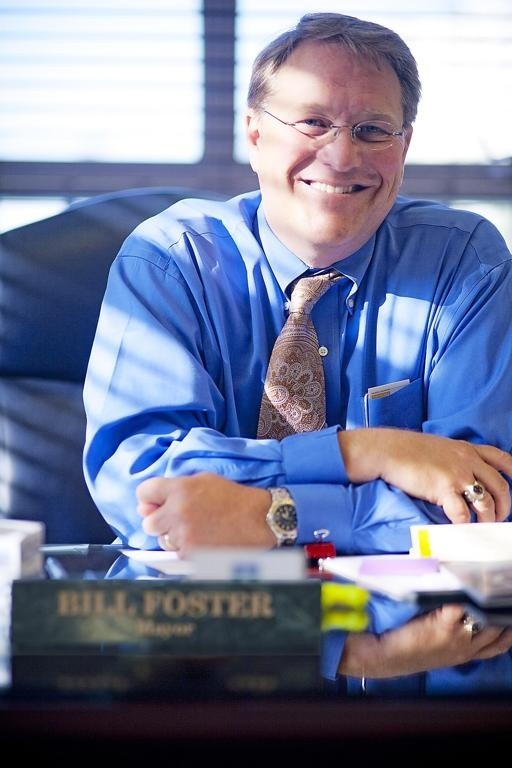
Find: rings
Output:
[464,481,485,503]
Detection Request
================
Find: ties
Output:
[257,272,349,439]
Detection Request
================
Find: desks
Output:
[1,536,512,766]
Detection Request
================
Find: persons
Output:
[83,12,512,554]
[320,599,512,681]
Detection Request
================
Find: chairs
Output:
[1,188,224,545]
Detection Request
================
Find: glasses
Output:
[264,110,406,151]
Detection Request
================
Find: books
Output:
[11,579,321,702]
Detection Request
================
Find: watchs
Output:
[265,488,298,550]
